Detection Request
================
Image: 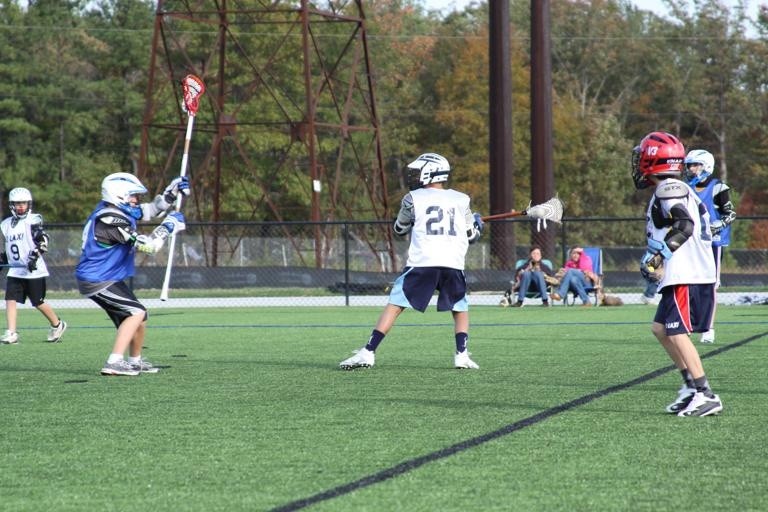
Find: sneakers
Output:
[455,348,479,369]
[678,392,723,416]
[513,302,524,307]
[700,328,714,343]
[47,320,67,343]
[0,330,19,344]
[543,302,549,307]
[127,361,159,373]
[101,359,140,375]
[340,348,375,369]
[666,384,697,413]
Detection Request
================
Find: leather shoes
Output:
[581,304,592,307]
[550,294,560,300]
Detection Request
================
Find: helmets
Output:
[407,153,450,190]
[684,149,715,186]
[9,187,32,219]
[632,132,685,189]
[101,172,147,220]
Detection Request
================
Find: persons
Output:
[550,247,594,307]
[684,149,736,343]
[512,246,554,308]
[0,188,67,344]
[632,131,723,417]
[76,172,190,376]
[338,153,485,368]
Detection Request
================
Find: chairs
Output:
[510,260,554,307]
[558,247,604,307]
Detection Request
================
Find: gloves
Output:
[640,238,673,283]
[472,213,484,232]
[163,176,190,203]
[710,220,724,236]
[28,256,37,272]
[161,210,186,236]
